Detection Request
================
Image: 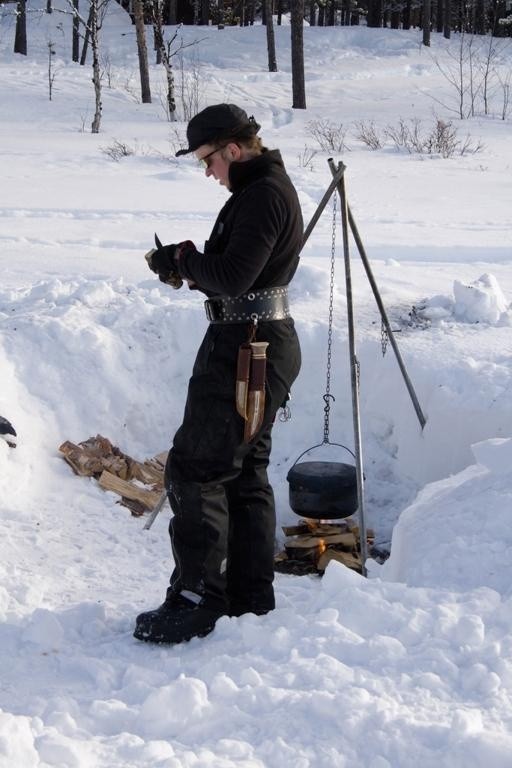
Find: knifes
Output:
[236,341,269,442]
[154,233,163,250]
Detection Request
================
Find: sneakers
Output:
[133,567,275,644]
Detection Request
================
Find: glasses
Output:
[199,141,242,169]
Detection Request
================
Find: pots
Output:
[287,440,366,520]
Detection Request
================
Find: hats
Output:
[175,103,249,157]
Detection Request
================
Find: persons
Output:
[133,103,305,643]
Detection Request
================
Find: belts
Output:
[204,286,290,323]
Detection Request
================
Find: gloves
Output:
[144,232,184,290]
